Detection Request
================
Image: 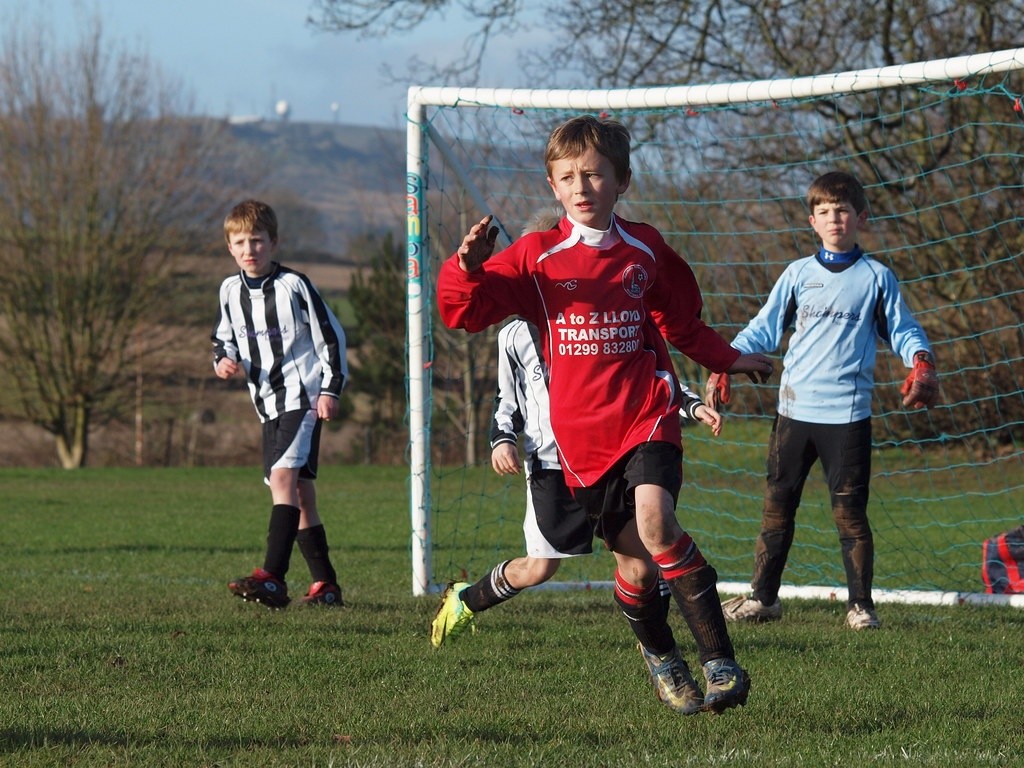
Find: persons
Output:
[706,171,938,629]
[209,196,349,607]
[437,113,777,715]
[430,206,723,651]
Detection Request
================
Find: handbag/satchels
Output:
[981,524,1024,595]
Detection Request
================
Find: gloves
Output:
[900,352,941,411]
[704,372,730,413]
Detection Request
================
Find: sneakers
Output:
[227,568,292,611]
[430,581,476,649]
[702,658,750,715]
[844,603,881,631]
[295,581,344,606]
[637,641,704,716]
[720,593,782,623]
